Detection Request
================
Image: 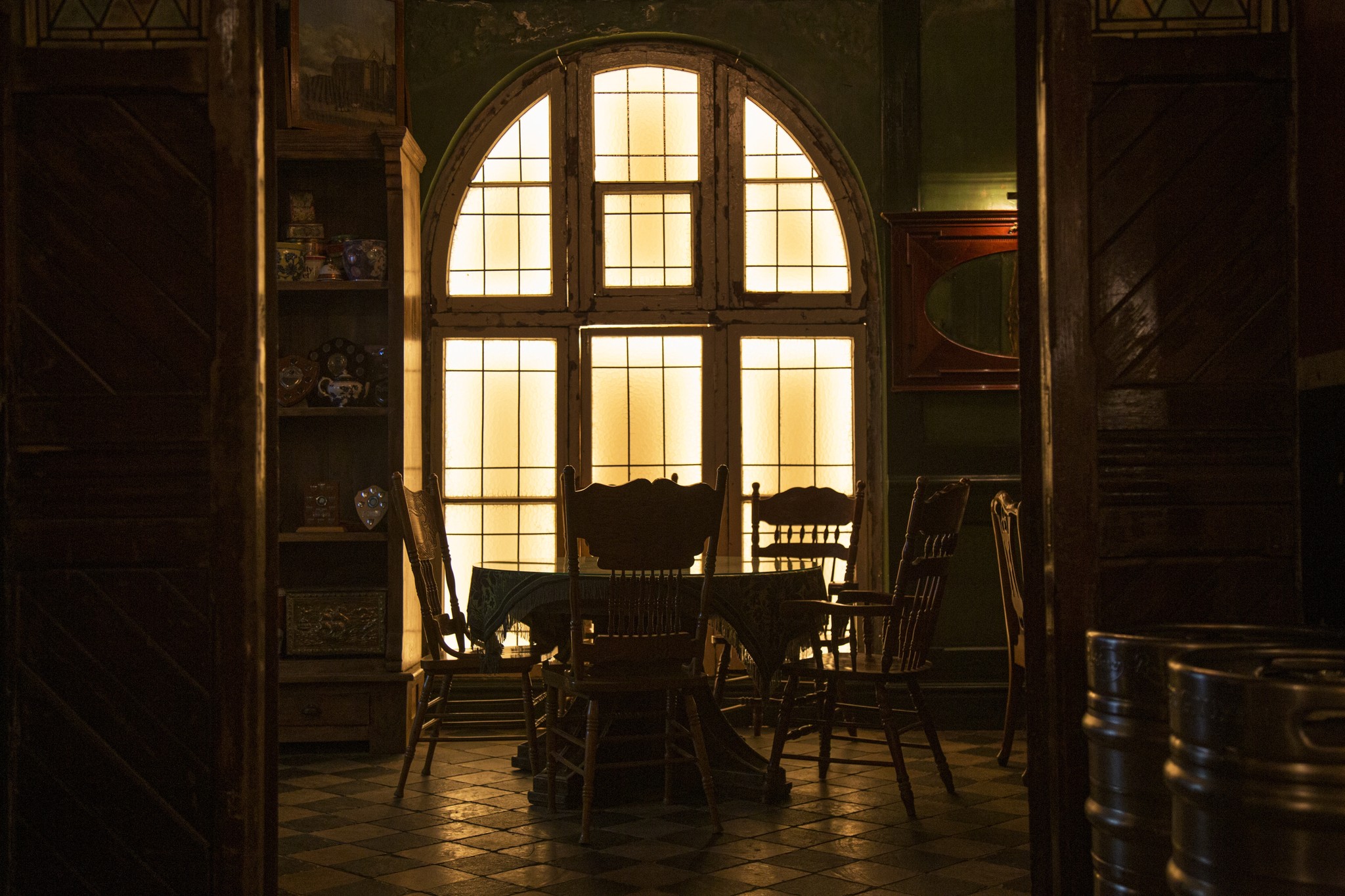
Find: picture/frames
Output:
[289,0,408,132]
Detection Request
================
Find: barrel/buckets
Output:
[1163,646,1345,896]
[1081,623,1335,896]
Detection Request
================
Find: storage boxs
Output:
[283,586,387,657]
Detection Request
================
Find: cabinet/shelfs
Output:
[265,129,401,749]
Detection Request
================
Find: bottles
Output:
[343,240,388,281]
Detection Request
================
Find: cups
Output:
[277,242,307,281]
[300,255,326,282]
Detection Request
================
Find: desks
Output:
[467,560,833,810]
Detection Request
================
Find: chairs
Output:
[387,459,1023,845]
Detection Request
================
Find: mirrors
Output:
[881,209,1019,392]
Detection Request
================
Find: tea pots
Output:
[318,370,370,407]
